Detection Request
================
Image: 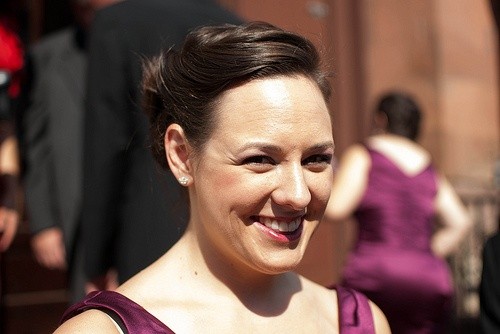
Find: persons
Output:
[1,0,393,334]
[325,87,472,334]
